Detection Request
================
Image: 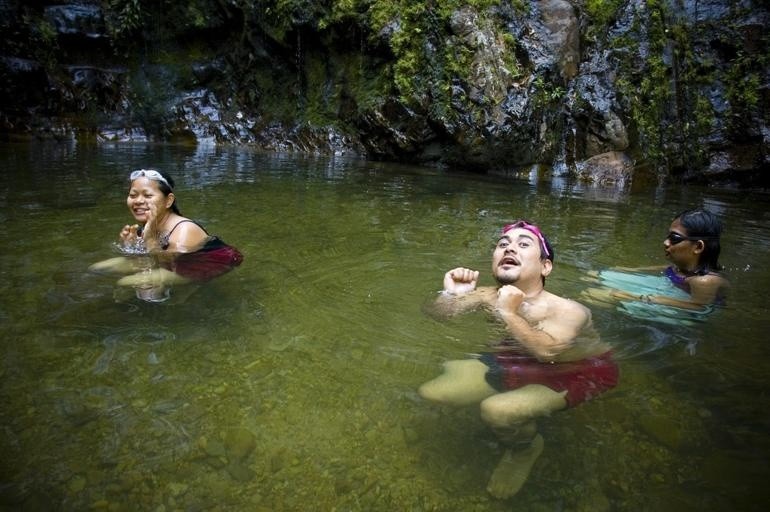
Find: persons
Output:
[86,166,243,306]
[417,219,619,500]
[581,207,732,346]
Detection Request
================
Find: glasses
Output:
[129,169,175,192]
[667,235,702,244]
[504,221,550,256]
